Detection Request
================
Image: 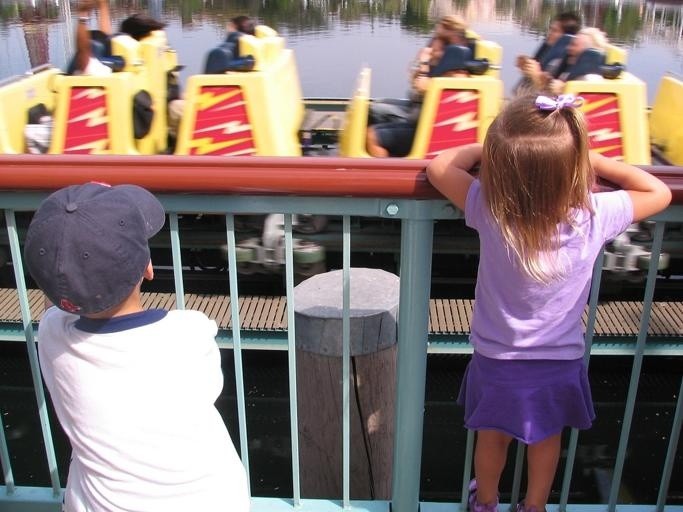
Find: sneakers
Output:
[467,477,499,512]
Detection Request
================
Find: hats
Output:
[22,181,167,316]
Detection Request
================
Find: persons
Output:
[366,14,610,156]
[23,181,250,512]
[22,2,256,161]
[425,90,672,511]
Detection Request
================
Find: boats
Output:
[0,30,681,295]
